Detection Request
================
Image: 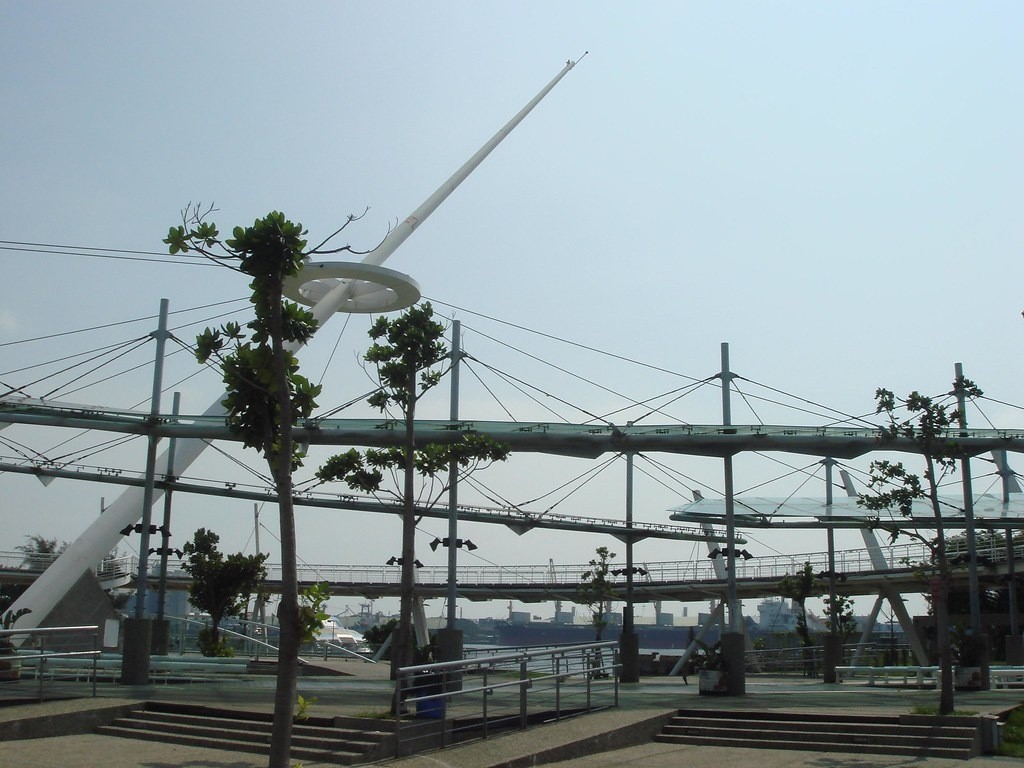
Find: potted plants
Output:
[0,608,32,681]
[682,635,725,696]
[950,623,991,691]
